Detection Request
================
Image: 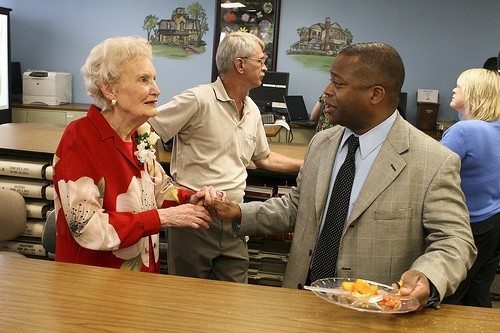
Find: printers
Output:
[22,70,73,106]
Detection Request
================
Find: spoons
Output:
[304,285,383,303]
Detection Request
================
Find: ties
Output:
[310,135,360,284]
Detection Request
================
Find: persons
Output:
[196,42,478,309]
[310,94,335,133]
[55,36,227,274]
[440,67,500,308]
[148,31,304,284]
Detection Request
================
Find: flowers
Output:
[133,122,161,166]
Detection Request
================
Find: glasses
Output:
[241,56,266,66]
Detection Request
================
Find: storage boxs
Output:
[21,70,73,106]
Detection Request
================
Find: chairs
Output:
[0,189,27,241]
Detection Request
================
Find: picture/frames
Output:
[211,0,281,83]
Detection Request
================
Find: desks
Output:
[0,254,500,333]
[262,119,287,142]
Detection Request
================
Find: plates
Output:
[310,277,420,315]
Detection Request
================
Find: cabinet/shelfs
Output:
[0,103,315,285]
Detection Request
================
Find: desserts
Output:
[342,278,378,307]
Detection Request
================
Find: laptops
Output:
[283,94,316,127]
